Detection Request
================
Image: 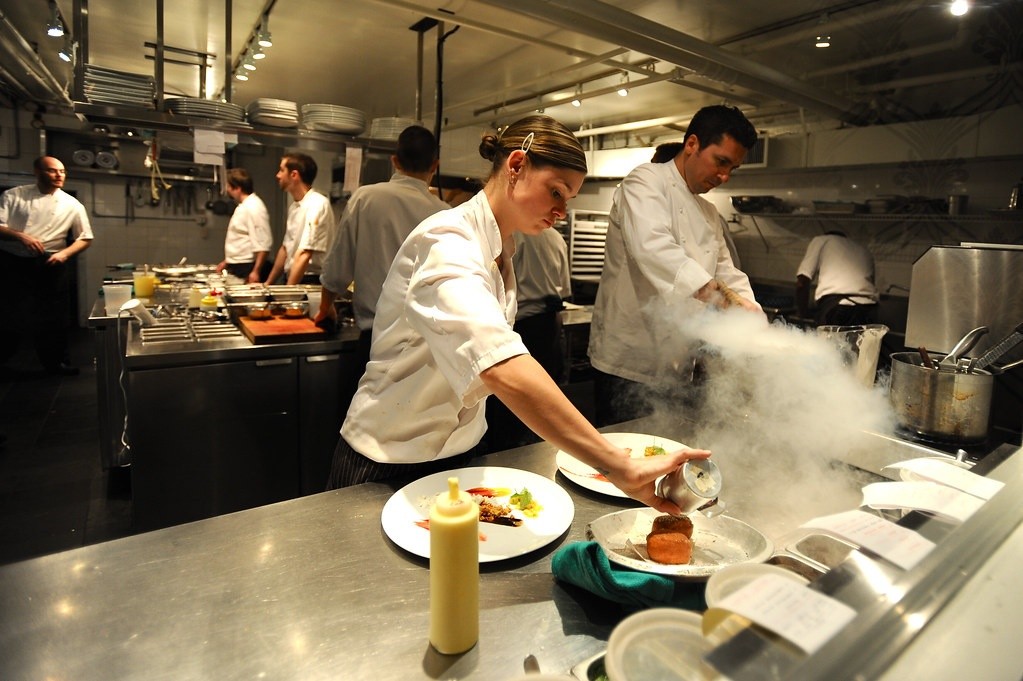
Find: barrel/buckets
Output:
[103,285,133,318]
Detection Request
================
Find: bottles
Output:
[189,285,203,308]
[949,180,969,214]
[429,477,479,655]
[200,293,217,313]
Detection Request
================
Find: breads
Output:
[646,513,693,564]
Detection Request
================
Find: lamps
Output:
[235,58,249,83]
[257,12,274,49]
[251,37,267,61]
[815,13,833,50]
[244,41,258,71]
[617,70,630,97]
[55,36,78,61]
[490,107,498,129]
[571,81,583,107]
[535,94,545,114]
[44,2,65,39]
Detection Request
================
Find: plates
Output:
[300,104,367,135]
[370,118,425,141]
[382,466,575,563]
[82,63,155,109]
[589,507,774,576]
[166,97,245,122]
[556,432,694,497]
[247,97,299,127]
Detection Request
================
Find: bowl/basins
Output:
[228,283,310,327]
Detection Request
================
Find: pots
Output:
[890,351,994,443]
[729,194,783,213]
[105,263,135,271]
[155,261,216,276]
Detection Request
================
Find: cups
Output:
[132,272,156,297]
[307,292,321,319]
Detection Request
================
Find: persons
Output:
[505,227,572,445]
[795,231,880,325]
[314,126,453,354]
[586,105,769,428]
[324,116,712,518]
[264,153,335,285]
[216,169,273,283]
[0,156,95,380]
